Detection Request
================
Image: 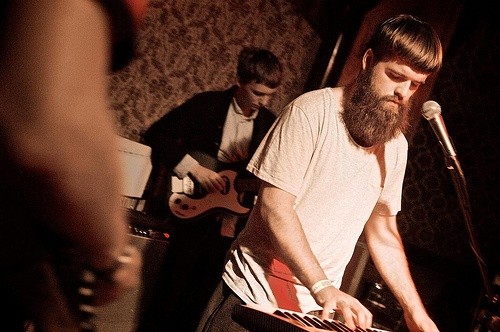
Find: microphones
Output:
[421,100,465,183]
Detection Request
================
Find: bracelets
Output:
[112,244,137,270]
[310,279,332,296]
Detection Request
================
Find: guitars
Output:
[169,169,267,221]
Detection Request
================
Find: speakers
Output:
[91,208,177,332]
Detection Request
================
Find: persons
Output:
[198,14,441,332]
[0,0,147,332]
[136,46,285,332]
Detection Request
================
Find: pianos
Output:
[233,303,397,332]
